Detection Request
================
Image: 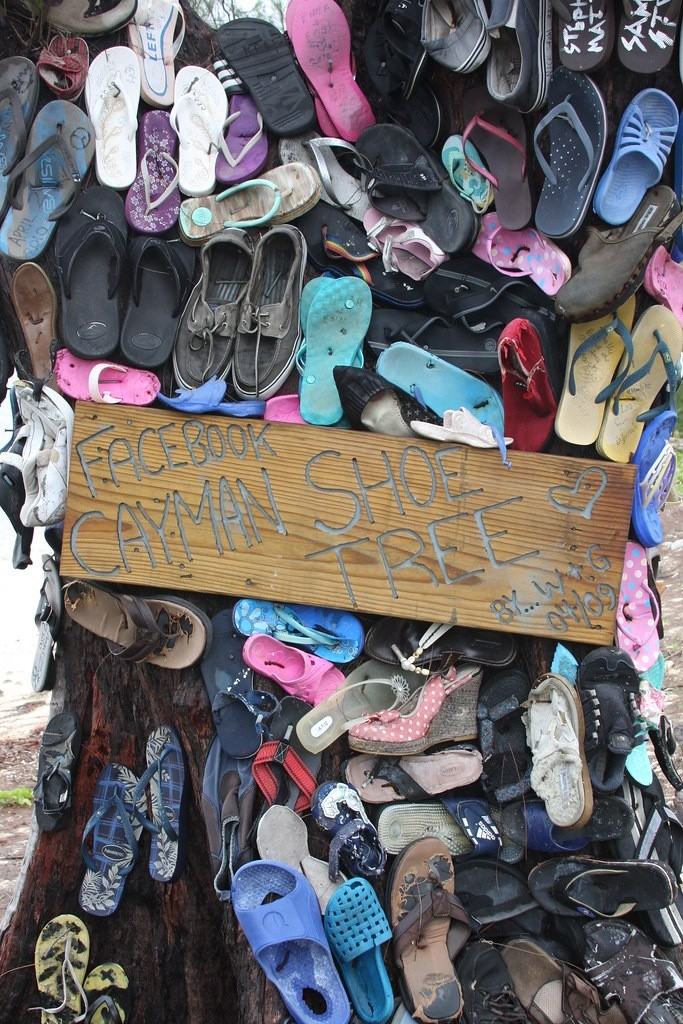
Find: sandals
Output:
[83,964,131,1024]
[32,914,90,1024]
[32,713,82,831]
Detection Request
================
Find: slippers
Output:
[202,543,683,1024]
[64,580,211,667]
[0,0,683,528]
[78,763,145,917]
[133,725,184,882]
[34,556,63,642]
